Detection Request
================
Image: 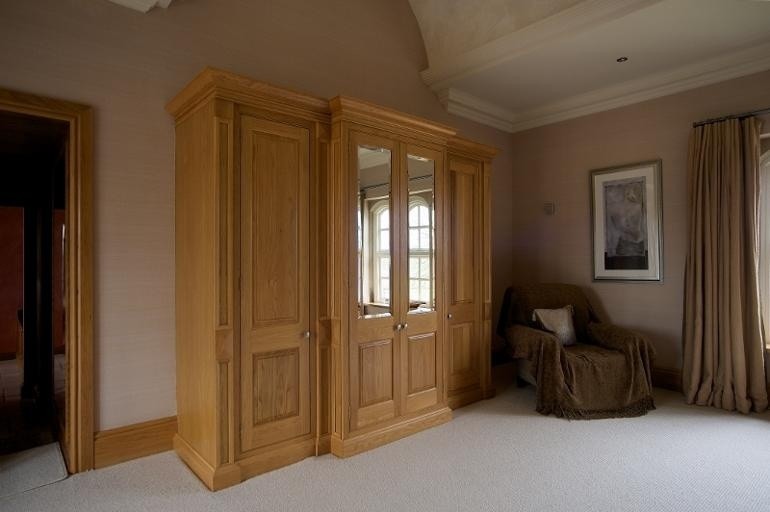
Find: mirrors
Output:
[357,140,438,319]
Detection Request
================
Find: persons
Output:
[610,182,643,255]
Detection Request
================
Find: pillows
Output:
[532,304,577,347]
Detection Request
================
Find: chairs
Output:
[495,283,653,420]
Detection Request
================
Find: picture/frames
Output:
[588,157,664,285]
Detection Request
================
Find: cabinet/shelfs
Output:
[163,67,501,492]
